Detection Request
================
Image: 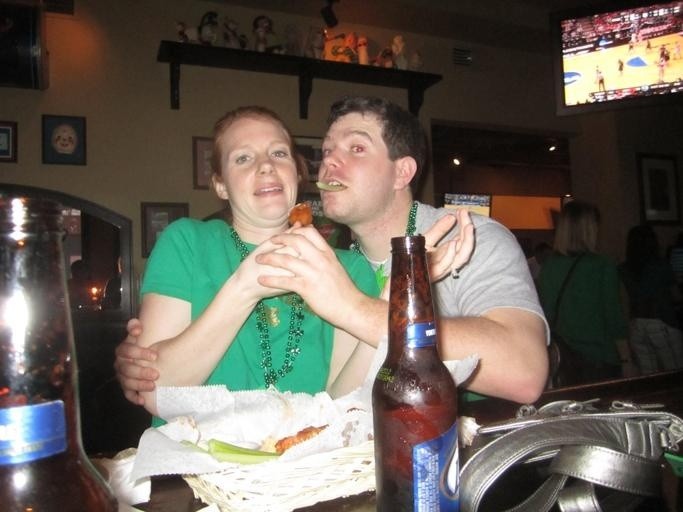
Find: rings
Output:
[452,268,460,279]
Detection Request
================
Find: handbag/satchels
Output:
[456,399,682,511]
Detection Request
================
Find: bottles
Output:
[1,193,121,512]
[370,234,465,512]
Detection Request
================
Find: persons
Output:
[615,223,682,374]
[563,2,682,106]
[109,92,555,429]
[532,198,642,384]
[130,104,381,432]
[524,240,550,284]
[668,229,682,286]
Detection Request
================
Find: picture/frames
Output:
[636,152,680,228]
[0,120,18,162]
[41,114,87,165]
[141,202,189,258]
[291,136,324,195]
[192,136,216,191]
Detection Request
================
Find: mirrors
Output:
[1,183,131,321]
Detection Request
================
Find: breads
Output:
[288,203,313,227]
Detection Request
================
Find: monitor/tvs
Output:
[550,0,683,117]
[442,192,493,218]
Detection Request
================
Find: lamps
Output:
[321,0,338,27]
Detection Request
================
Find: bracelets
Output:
[619,357,636,365]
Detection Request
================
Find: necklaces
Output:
[227,224,307,390]
[351,197,420,280]
